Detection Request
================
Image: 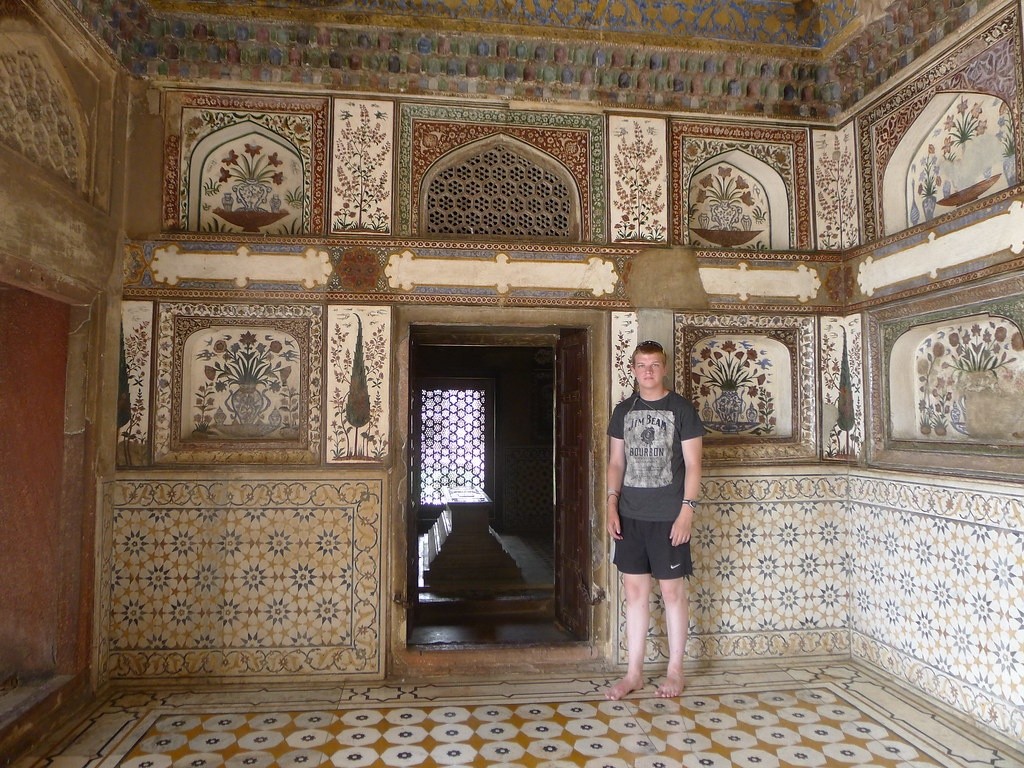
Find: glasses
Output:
[636,340,665,355]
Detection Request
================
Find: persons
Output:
[604,340,708,700]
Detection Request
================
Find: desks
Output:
[424,485,524,582]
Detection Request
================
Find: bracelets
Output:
[607,488,619,501]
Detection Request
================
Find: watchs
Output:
[682,500,696,508]
